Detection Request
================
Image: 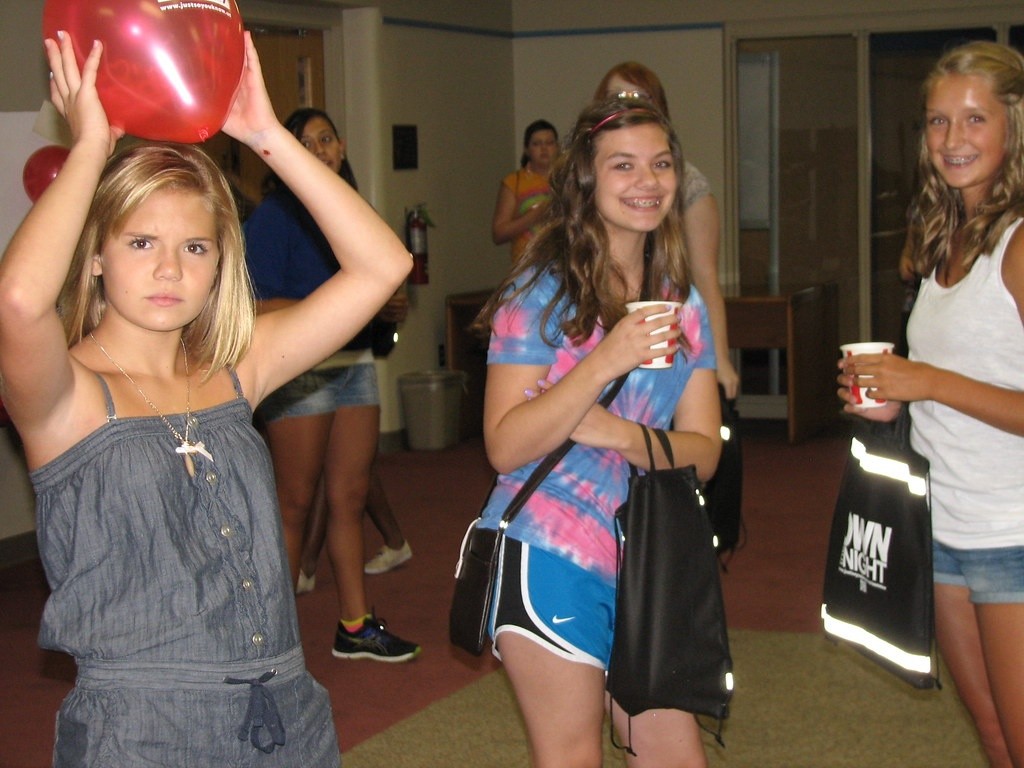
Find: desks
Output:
[717,281,831,445]
[448,283,501,384]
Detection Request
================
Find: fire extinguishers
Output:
[405,203,429,283]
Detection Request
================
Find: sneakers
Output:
[332,618,422,662]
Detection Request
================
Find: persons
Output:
[241,107,423,664]
[837,41,1024,768]
[491,120,564,265]
[447,93,720,768]
[1,28,414,768]
[593,61,741,549]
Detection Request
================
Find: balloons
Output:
[42,0,246,144]
[23,144,70,204]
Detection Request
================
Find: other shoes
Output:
[295,569,316,593]
[364,539,412,575]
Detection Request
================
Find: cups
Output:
[839,342,895,408]
[387,277,408,306]
[625,301,683,369]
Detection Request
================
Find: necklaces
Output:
[943,254,980,287]
[90,330,215,476]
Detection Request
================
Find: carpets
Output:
[340,626,992,768]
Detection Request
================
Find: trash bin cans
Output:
[397,370,469,452]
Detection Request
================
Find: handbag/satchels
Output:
[605,423,735,756]
[703,383,748,575]
[821,401,941,689]
[450,527,504,657]
